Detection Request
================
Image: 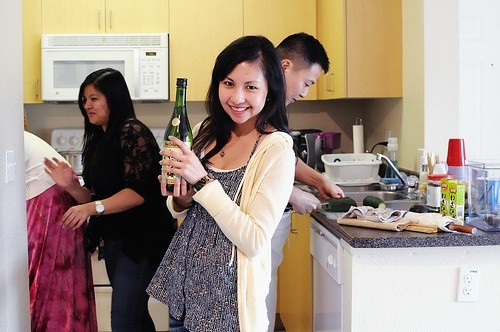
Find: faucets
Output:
[375,153,418,193]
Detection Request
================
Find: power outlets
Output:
[456,267,477,303]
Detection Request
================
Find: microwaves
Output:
[40,34,169,103]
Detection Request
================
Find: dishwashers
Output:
[309,217,343,332]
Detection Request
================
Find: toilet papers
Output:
[351,125,365,154]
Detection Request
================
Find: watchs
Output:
[94,200,104,217]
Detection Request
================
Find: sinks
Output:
[317,193,423,205]
[296,183,387,192]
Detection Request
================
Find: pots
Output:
[58,151,84,176]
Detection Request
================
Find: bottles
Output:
[165,78,192,192]
[427,174,454,211]
[384,137,399,179]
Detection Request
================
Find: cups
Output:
[484,178,500,227]
[445,138,467,167]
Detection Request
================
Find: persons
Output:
[145,35,296,332]
[267,32,345,332]
[45,68,178,332]
[23,131,98,332]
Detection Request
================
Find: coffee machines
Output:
[290,129,323,165]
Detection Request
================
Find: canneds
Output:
[426,173,454,211]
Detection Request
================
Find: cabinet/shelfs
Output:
[24,0,169,105]
[169,0,316,101]
[316,0,402,100]
[280,209,313,332]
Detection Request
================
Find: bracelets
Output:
[191,173,216,192]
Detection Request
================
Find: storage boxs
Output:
[321,152,382,180]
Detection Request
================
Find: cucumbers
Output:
[324,197,358,212]
[363,195,386,209]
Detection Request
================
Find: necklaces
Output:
[221,131,241,156]
